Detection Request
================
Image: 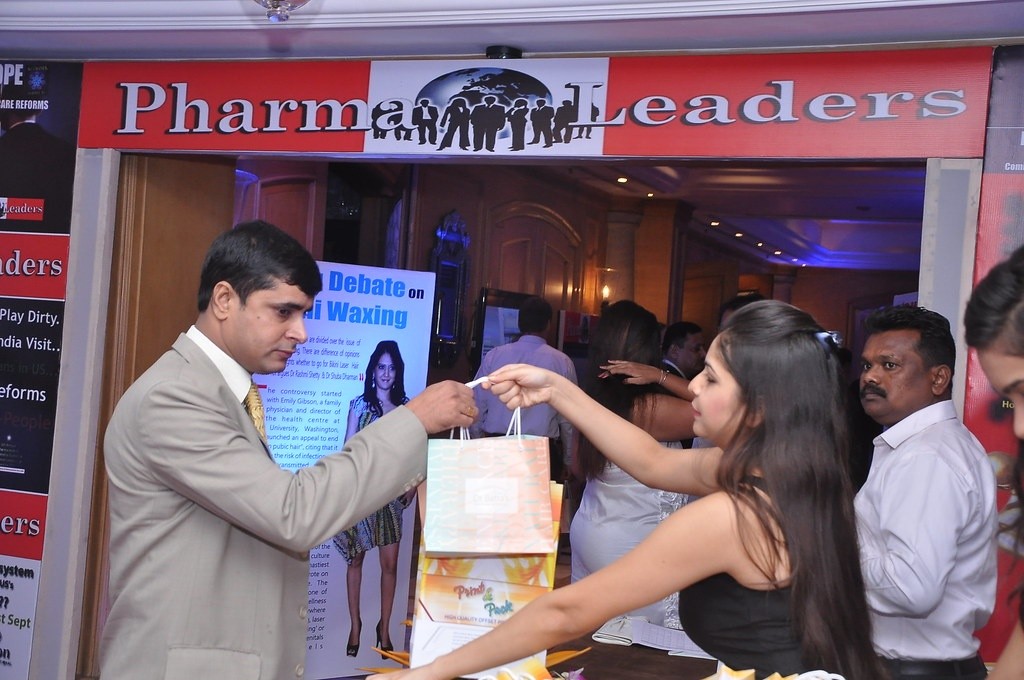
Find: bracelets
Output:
[658,369,669,385]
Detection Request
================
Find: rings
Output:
[466,405,476,416]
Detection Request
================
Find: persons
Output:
[98,219,480,680]
[366,245,1024,680]
[332,340,416,659]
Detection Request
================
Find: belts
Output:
[879,656,987,676]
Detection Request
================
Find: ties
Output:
[244,379,267,444]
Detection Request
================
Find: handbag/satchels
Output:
[410,479,564,680]
[424,377,557,561]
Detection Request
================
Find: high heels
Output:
[375,620,394,660]
[346,618,362,657]
